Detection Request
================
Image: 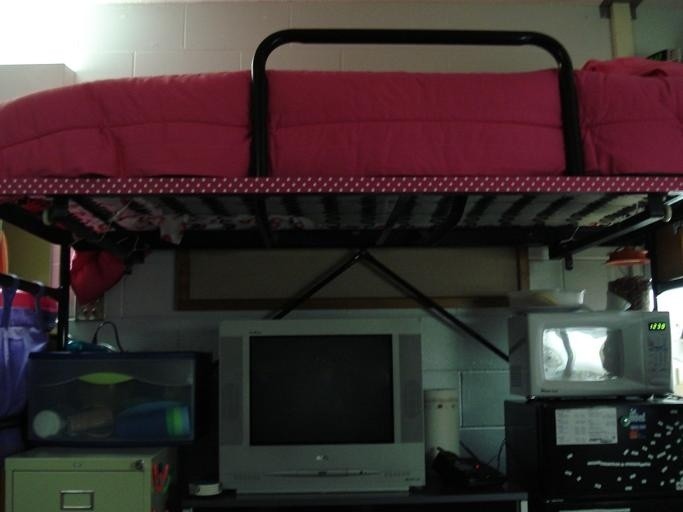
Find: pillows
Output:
[582,57,683,77]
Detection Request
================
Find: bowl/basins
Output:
[505,287,587,313]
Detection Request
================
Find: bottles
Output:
[605,248,653,312]
[424,388,462,458]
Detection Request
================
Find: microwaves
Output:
[507,310,673,401]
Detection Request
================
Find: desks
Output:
[183,486,529,512]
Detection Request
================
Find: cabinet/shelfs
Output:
[5,445,181,512]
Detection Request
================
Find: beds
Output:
[0,28,683,260]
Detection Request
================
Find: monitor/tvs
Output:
[217,318,426,497]
